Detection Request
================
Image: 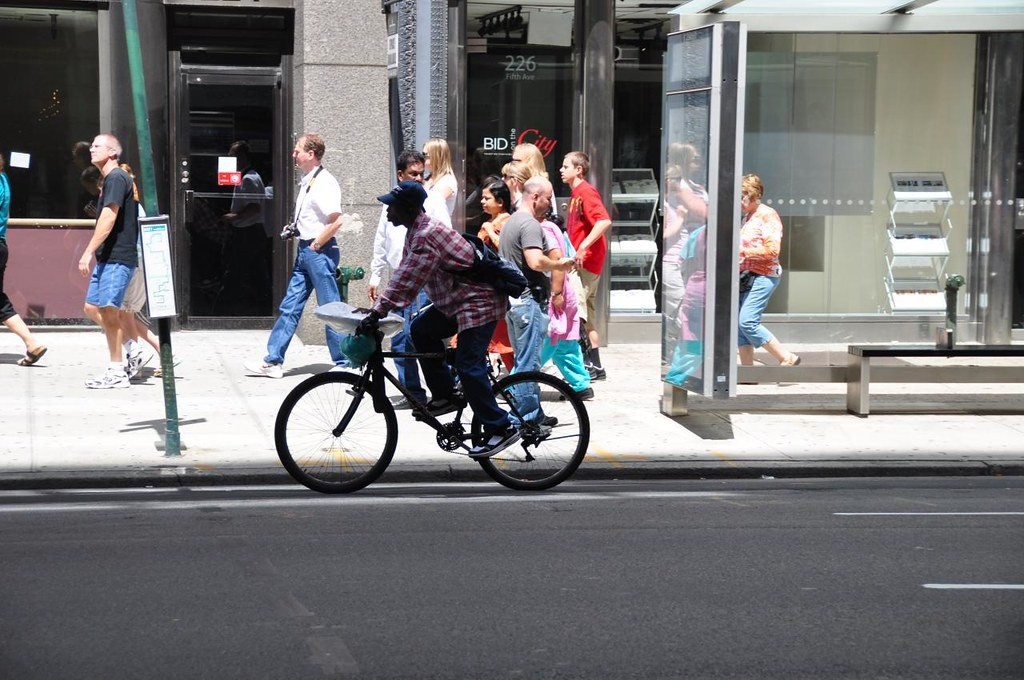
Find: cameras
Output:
[280,224,300,241]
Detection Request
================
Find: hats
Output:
[377,179,427,213]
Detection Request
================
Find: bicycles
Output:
[273,299,588,496]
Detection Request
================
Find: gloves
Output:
[360,310,379,334]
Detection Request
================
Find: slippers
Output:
[18,344,48,367]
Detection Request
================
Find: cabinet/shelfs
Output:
[611,169,659,315]
[881,173,953,316]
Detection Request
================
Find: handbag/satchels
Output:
[515,259,552,304]
[436,232,529,299]
[737,269,757,293]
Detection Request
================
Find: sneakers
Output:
[584,361,597,380]
[124,349,155,379]
[84,367,130,389]
[391,396,413,410]
[328,365,365,377]
[412,391,467,417]
[242,358,284,379]
[590,365,607,381]
[468,422,522,459]
[154,352,183,377]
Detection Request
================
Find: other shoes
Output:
[530,413,559,427]
[779,354,801,366]
[559,387,595,401]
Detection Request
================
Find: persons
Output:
[375,181,520,456]
[477,142,594,399]
[224,139,271,303]
[422,138,457,229]
[739,173,800,385]
[559,152,612,381]
[0,151,46,366]
[367,149,452,410]
[77,133,183,389]
[243,134,365,379]
[658,143,707,388]
[68,141,94,219]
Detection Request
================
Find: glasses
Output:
[504,174,513,180]
[422,151,428,158]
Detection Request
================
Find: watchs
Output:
[312,239,321,249]
[550,289,562,295]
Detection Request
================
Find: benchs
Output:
[848,343,1024,416]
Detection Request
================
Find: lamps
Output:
[616,23,668,53]
[476,5,524,36]
